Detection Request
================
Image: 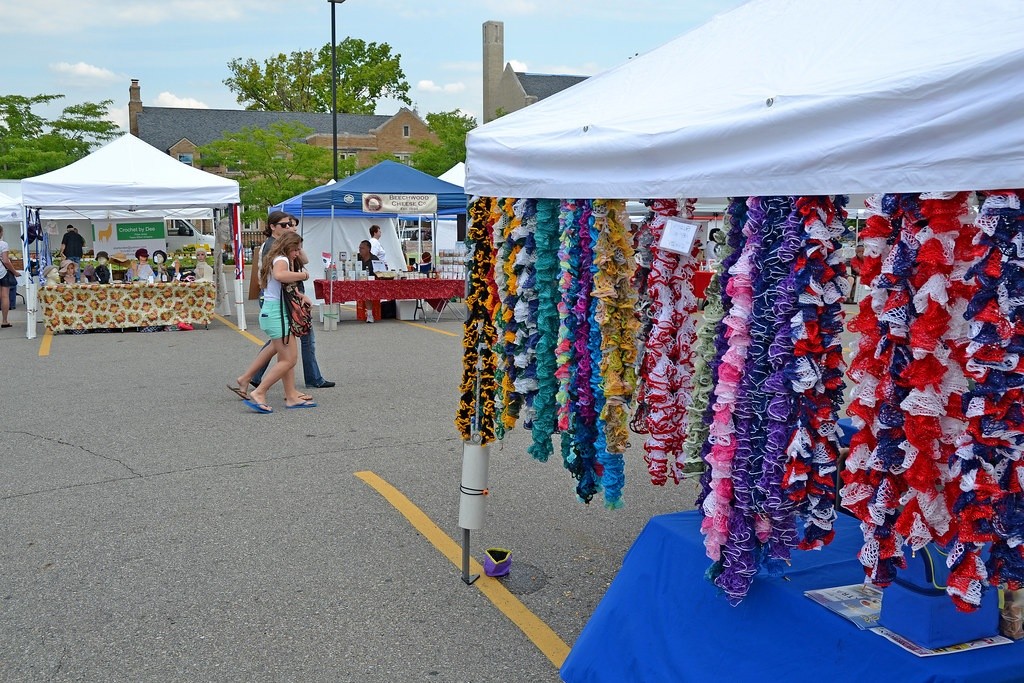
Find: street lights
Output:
[328,0,346,181]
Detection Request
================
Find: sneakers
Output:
[367,315,374,323]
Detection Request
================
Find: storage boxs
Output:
[394,298,419,320]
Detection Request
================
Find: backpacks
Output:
[271,258,312,338]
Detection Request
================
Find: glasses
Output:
[275,221,293,228]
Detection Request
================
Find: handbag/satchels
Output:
[20,210,38,245]
[6,270,18,286]
[35,210,43,241]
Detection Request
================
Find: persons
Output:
[357,240,380,280]
[367,224,390,272]
[93,251,110,284]
[44,265,60,286]
[193,248,214,282]
[60,224,86,283]
[128,249,162,281]
[0,225,21,328]
[59,260,79,285]
[226,211,336,414]
[422,252,431,263]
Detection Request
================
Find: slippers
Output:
[285,402,317,408]
[243,399,273,414]
[226,384,250,400]
[285,393,313,400]
[1,323,12,328]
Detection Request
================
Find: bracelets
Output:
[302,272,307,281]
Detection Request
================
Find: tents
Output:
[21,132,248,339]
[0,179,221,235]
[458,0,1024,587]
[266,158,467,332]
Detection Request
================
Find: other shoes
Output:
[249,380,260,388]
[312,381,336,388]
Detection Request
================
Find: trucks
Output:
[165,219,216,255]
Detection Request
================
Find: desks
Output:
[314,279,466,323]
[38,281,216,332]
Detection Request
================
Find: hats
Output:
[153,250,167,265]
[95,251,109,261]
[179,270,196,282]
[58,259,78,276]
[196,247,207,258]
[94,264,110,283]
[83,265,95,276]
[67,224,73,229]
[111,252,130,265]
[135,248,149,258]
[43,265,59,278]
